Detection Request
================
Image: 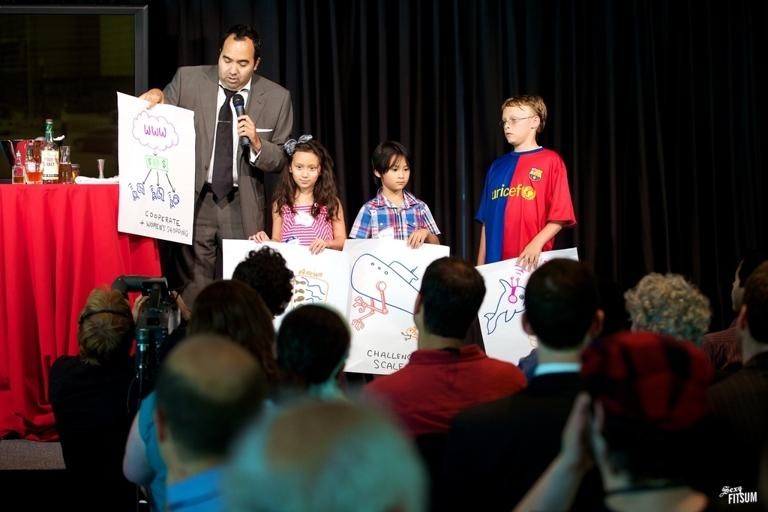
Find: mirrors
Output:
[1,6,148,180]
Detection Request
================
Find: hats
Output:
[580,333,713,425]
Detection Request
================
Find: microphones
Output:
[233,94,251,146]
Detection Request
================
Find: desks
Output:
[0,181,162,444]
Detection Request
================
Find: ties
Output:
[212,85,238,201]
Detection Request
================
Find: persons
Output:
[473,93,579,273]
[248,133,346,255]
[45,244,767,512]
[137,27,295,313]
[347,138,442,249]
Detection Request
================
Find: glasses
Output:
[499,113,533,129]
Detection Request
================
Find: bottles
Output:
[12,118,78,185]
[96,158,106,179]
